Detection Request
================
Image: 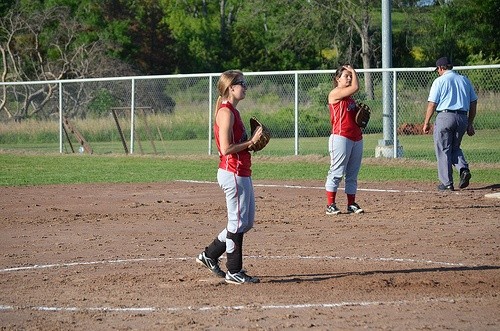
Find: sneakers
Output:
[196,252,225,278]
[326,203,341,215]
[348,202,363,214]
[225,269,260,285]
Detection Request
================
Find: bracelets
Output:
[424,123,429,125]
[248,139,255,145]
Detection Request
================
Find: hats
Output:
[434,57,451,72]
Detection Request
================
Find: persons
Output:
[422,56,478,192]
[195,70,263,284]
[324,65,365,215]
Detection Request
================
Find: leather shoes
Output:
[437,183,454,191]
[458,170,470,189]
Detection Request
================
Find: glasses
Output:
[233,81,246,86]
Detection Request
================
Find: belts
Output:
[438,110,468,115]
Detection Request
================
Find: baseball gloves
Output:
[355,103,371,128]
[248,116,270,151]
